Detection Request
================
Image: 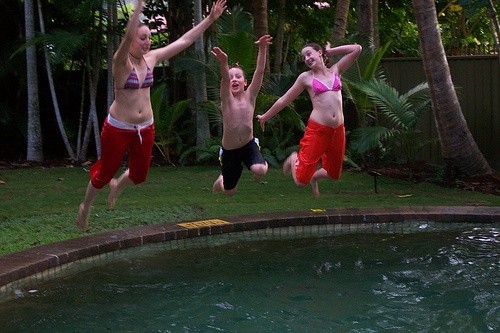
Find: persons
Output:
[256,41,361,197]
[76,1,228,231]
[208,35,274,195]
